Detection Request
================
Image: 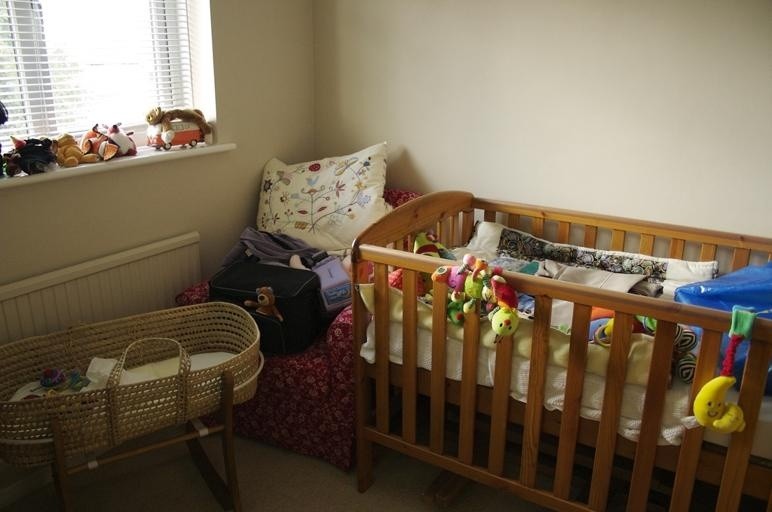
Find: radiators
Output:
[0,229,203,349]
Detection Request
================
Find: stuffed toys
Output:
[0,100,214,177]
[682,305,760,440]
[587,299,661,348]
[412,228,548,344]
[244,284,284,321]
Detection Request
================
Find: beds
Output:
[345,188,771,511]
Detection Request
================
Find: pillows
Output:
[254,139,397,260]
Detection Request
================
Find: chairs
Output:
[174,187,425,477]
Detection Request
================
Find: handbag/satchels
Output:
[207,258,322,355]
